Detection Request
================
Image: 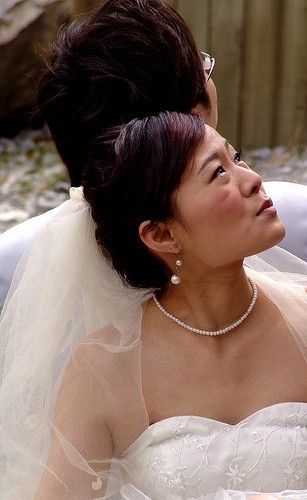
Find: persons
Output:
[0,0,306,315]
[0,110,306,500]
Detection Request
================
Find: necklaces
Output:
[152,274,257,336]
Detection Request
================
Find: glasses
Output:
[199,52,215,86]
